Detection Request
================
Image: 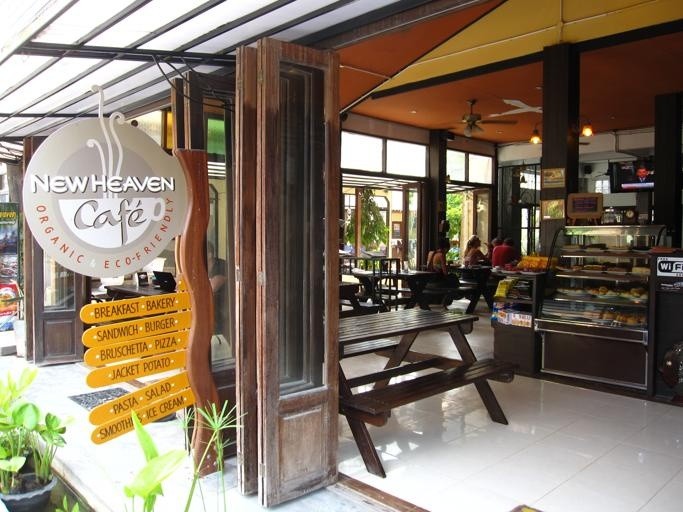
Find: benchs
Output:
[334,337,518,480]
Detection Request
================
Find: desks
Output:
[339,266,495,315]
[100,284,180,304]
[337,308,477,477]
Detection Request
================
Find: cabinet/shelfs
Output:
[491,271,547,378]
[532,221,670,391]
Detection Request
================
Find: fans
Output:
[437,99,518,132]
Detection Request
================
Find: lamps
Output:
[464,125,473,138]
[529,122,542,144]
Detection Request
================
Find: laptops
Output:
[154,271,176,291]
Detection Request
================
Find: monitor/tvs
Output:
[609,160,655,193]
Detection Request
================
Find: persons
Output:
[426,238,459,309]
[490,237,503,248]
[461,234,493,297]
[205,239,227,332]
[490,237,521,279]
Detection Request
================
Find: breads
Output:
[558,243,650,324]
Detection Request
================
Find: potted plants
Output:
[0,365,69,511]
